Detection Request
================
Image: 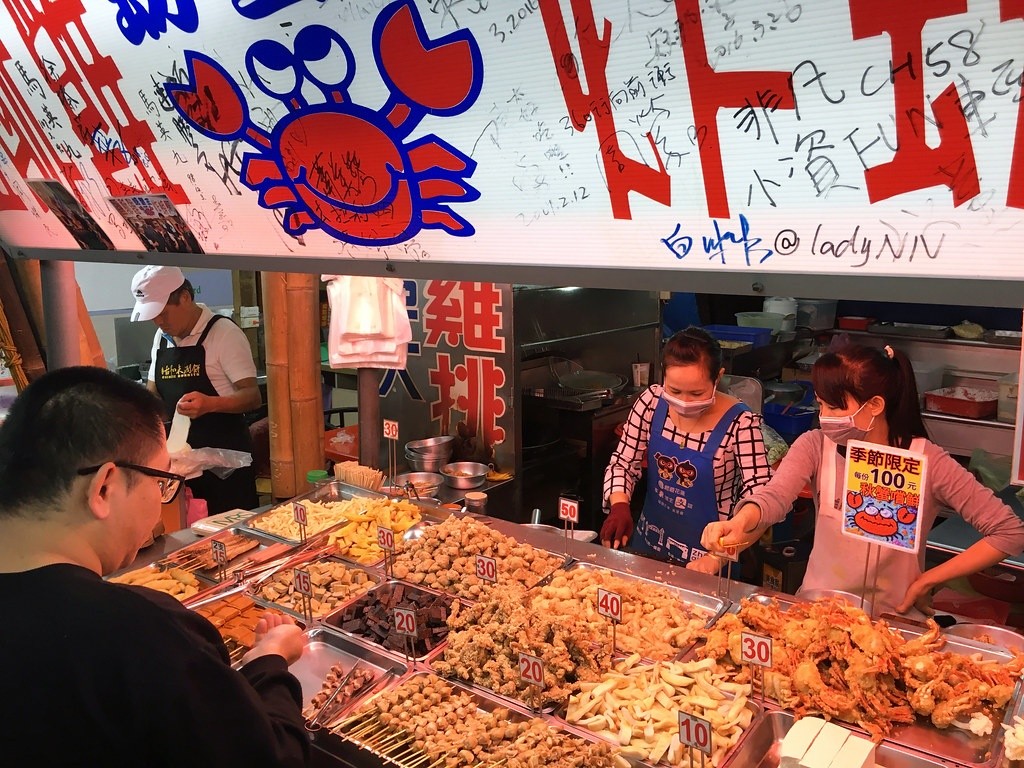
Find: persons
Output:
[128,265,262,513]
[0,366,316,768]
[599,326,772,585]
[701,342,1024,626]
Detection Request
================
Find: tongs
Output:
[405,480,420,501]
[304,657,395,731]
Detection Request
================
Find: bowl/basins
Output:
[837,316,876,330]
[439,461,491,490]
[925,385,999,421]
[734,312,785,335]
[392,472,445,498]
[797,299,839,330]
[940,623,1024,654]
[381,486,410,497]
[795,590,875,618]
[411,497,441,508]
[910,362,943,407]
[405,435,455,473]
[764,382,808,404]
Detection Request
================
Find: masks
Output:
[661,391,715,418]
[819,401,875,446]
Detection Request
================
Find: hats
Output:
[130,264,185,322]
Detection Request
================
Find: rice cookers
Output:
[763,295,798,331]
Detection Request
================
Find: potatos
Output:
[326,501,421,564]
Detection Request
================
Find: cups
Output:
[465,492,488,515]
[632,362,650,387]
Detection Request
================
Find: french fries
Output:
[565,653,754,768]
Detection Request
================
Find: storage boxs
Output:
[231,269,263,370]
[702,325,773,349]
[797,299,839,332]
[921,385,999,420]
[765,403,815,437]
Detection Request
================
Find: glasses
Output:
[78,461,185,504]
